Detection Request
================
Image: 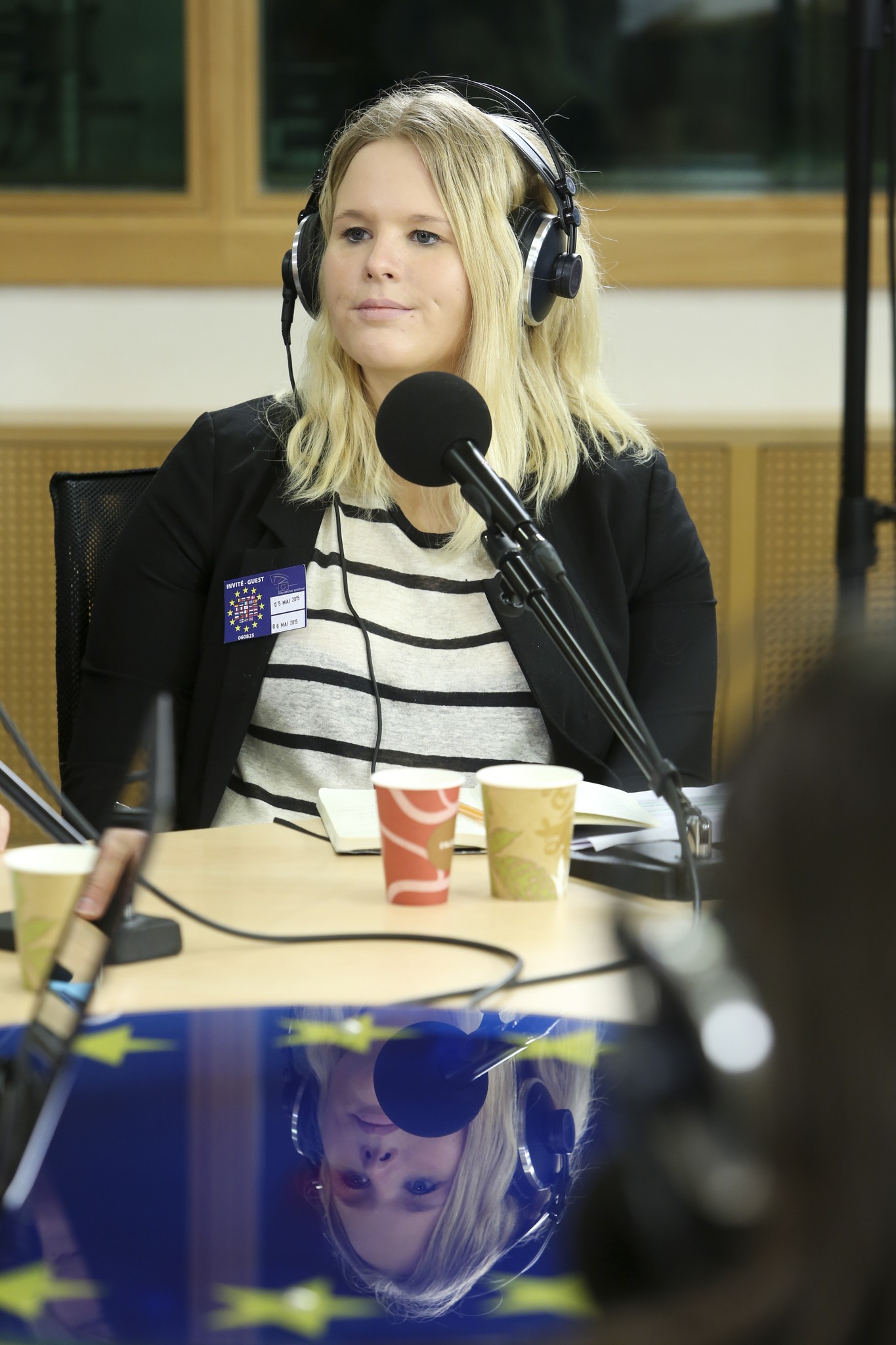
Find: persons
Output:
[289,1009,602,1322]
[69,71,727,840]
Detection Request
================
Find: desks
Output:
[0,804,716,1027]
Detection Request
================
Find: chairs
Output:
[39,464,160,833]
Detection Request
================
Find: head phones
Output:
[292,76,585,328]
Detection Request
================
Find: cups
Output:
[477,764,584,899]
[371,768,467,905]
[2,844,99,993]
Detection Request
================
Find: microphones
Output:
[371,1015,563,1139]
[376,372,566,578]
